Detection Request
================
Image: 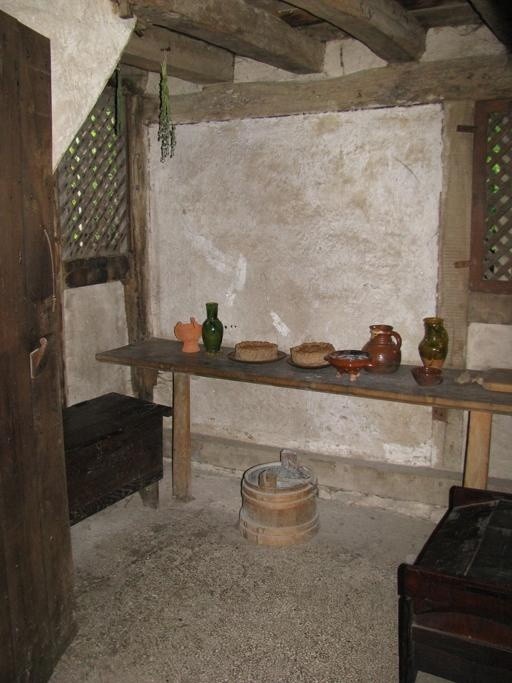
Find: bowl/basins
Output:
[411,367,440,385]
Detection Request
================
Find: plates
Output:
[226,350,336,369]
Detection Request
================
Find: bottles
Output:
[362,325,403,373]
[202,302,224,353]
[417,317,449,369]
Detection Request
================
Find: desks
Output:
[95,335,511,502]
